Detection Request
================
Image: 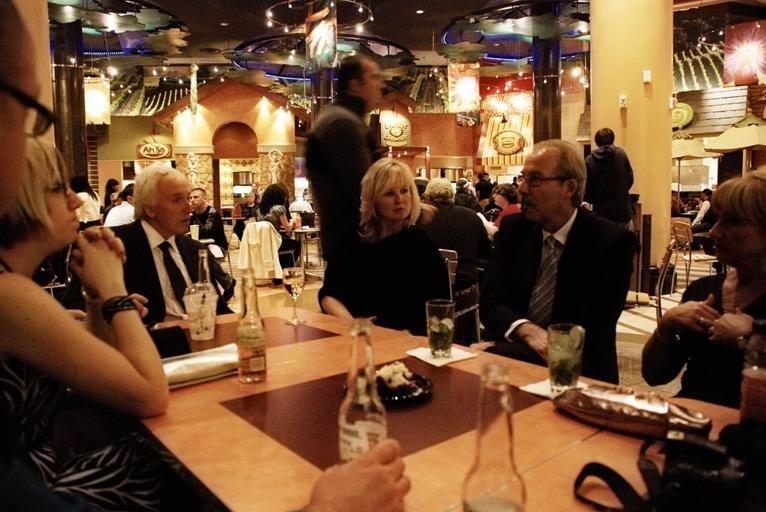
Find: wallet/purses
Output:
[149,325,191,358]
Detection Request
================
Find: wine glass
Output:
[283,267,309,327]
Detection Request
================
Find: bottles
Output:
[737,317,766,419]
[460,357,527,511]
[314,213,319,229]
[296,212,302,229]
[197,247,212,285]
[233,266,270,381]
[335,314,390,463]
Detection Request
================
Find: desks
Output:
[140,306,741,509]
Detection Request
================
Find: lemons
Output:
[569,326,584,350]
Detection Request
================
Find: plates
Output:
[340,368,435,410]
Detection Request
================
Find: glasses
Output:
[2,78,56,136]
[518,172,561,187]
[46,179,69,195]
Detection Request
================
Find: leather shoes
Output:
[223,278,235,301]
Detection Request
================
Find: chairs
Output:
[0,192,328,298]
[616,194,733,327]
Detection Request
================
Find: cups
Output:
[181,282,220,342]
[424,296,455,359]
[547,322,586,392]
[189,224,199,241]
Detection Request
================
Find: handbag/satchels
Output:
[573,429,765,512]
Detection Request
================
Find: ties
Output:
[159,239,188,315]
[528,235,559,330]
